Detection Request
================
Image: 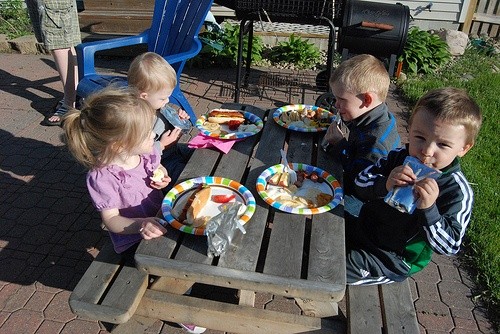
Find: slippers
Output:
[45,97,71,126]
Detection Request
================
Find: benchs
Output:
[68,237,148,324]
[347,277,422,334]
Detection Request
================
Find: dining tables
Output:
[134,103,346,302]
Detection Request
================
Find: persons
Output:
[35,0,82,124]
[62,52,208,334]
[329,52,481,286]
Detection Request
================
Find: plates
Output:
[195,110,263,140]
[162,177,256,236]
[257,163,344,215]
[273,104,336,132]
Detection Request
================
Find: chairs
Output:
[76,0,213,126]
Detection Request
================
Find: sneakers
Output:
[178,322,207,334]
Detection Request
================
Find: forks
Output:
[280,149,298,185]
